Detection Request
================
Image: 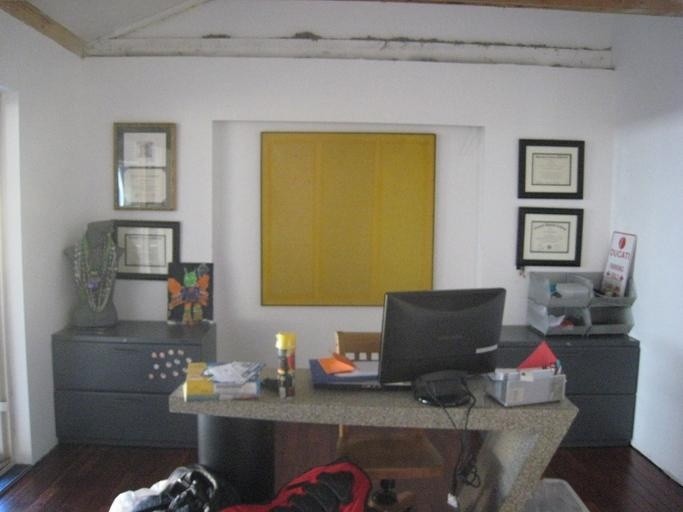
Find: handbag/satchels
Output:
[110,464,240,511]
[218,456,371,511]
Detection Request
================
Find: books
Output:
[181,359,261,403]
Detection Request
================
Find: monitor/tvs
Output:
[380,288,507,403]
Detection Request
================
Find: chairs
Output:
[333,331,444,512]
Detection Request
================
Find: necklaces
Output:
[73,233,117,312]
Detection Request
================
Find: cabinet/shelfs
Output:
[492,326,639,449]
[526,271,635,338]
[50,320,215,449]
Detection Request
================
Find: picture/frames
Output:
[111,120,177,211]
[108,219,179,281]
[513,137,584,269]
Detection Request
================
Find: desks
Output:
[167,368,578,512]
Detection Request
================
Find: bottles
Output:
[275,332,298,401]
